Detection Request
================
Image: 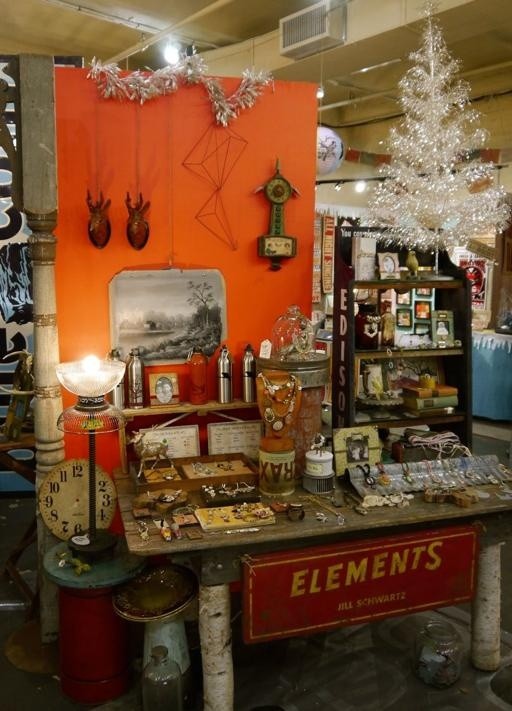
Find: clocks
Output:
[38,459,117,542]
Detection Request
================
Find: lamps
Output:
[55,354,129,562]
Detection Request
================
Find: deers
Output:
[86,190,111,245]
[132,431,175,477]
[124,192,150,246]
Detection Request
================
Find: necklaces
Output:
[349,455,507,500]
[256,372,299,432]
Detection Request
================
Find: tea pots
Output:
[362,363,384,400]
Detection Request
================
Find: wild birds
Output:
[406,250,418,280]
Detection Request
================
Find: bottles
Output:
[141,643,182,711]
[187,346,210,407]
[412,619,466,691]
[127,347,144,410]
[215,345,235,405]
[240,342,257,404]
[270,303,318,367]
[354,302,381,351]
[378,304,398,349]
[109,348,126,411]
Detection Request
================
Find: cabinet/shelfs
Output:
[337,225,473,445]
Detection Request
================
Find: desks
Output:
[113,449,512,709]
